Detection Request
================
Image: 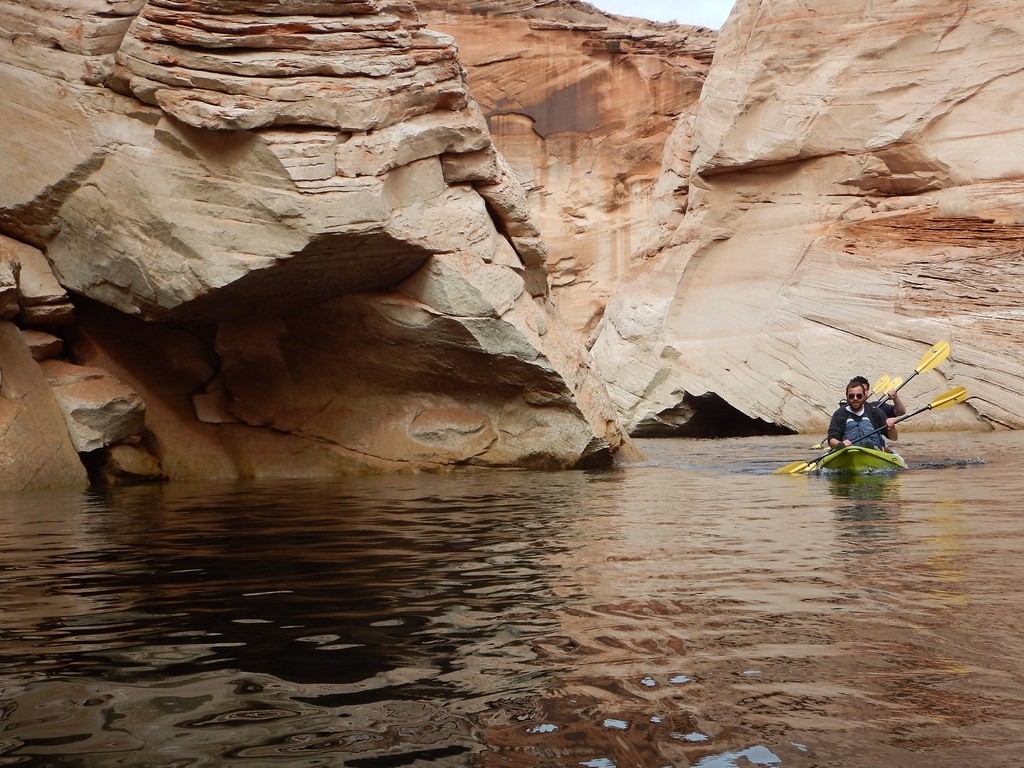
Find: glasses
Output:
[848,393,863,399]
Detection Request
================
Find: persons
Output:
[828,376,906,454]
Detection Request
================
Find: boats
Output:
[818,446,907,472]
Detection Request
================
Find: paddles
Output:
[826,339,952,456]
[811,375,902,452]
[773,385,969,475]
